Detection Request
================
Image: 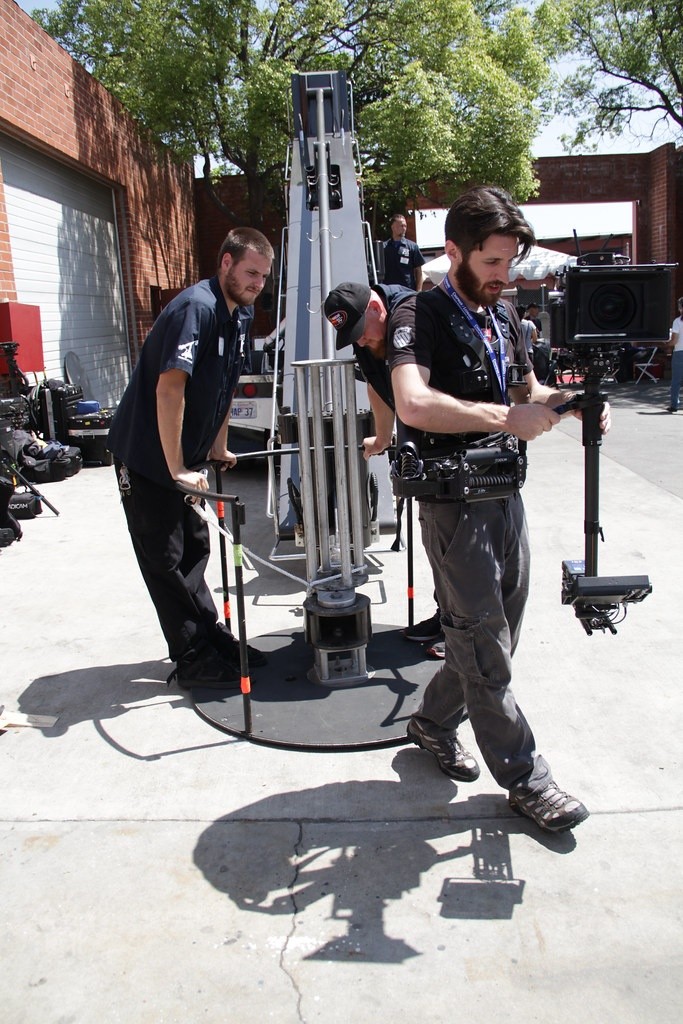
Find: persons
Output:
[513,303,543,370]
[323,283,447,660]
[658,293,683,417]
[385,185,612,836]
[380,213,427,292]
[107,227,275,689]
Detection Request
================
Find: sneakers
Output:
[427,642,447,659]
[407,720,481,782]
[404,612,443,640]
[508,781,588,832]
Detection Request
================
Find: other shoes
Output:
[667,407,677,413]
[219,640,267,668]
[176,652,255,688]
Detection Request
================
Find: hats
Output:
[527,302,541,309]
[323,282,372,350]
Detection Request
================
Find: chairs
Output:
[602,346,661,384]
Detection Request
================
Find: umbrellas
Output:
[415,244,591,303]
